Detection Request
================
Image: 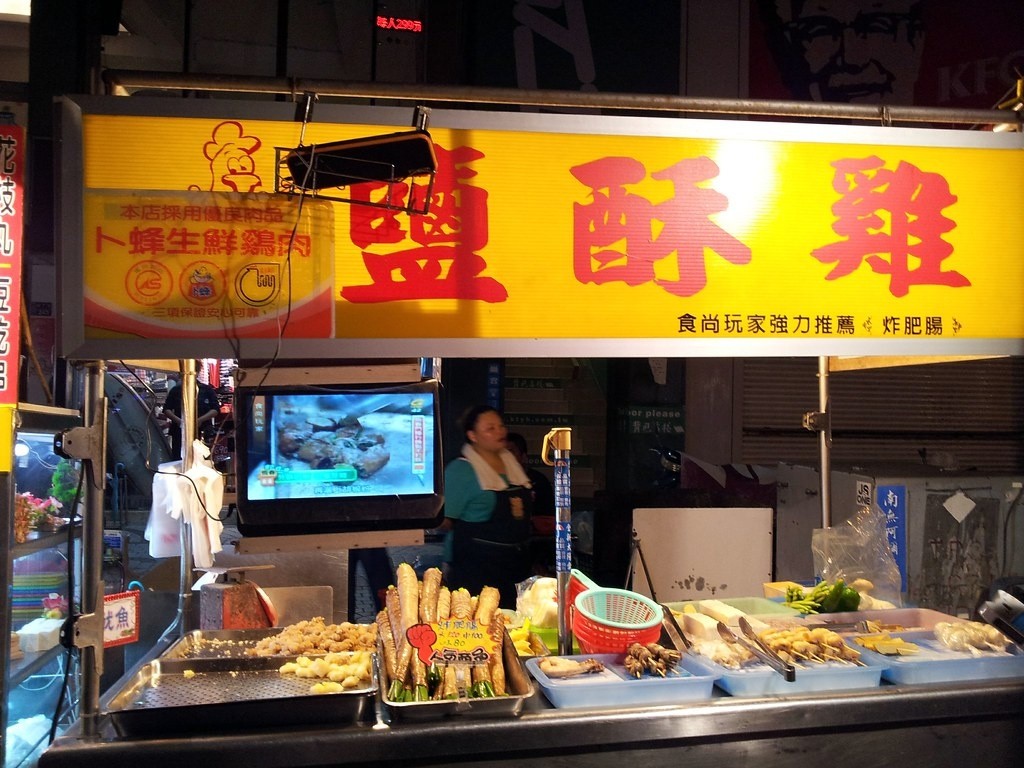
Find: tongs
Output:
[718,617,795,683]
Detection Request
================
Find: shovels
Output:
[635,536,689,653]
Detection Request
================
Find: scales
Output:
[192,562,280,629]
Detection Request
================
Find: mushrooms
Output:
[853,578,894,610]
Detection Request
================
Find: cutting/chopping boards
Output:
[275,408,432,499]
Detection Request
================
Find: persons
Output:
[438,405,539,611]
[162,359,220,461]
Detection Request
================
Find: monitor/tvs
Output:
[235,378,445,535]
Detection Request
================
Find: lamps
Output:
[275,92,437,192]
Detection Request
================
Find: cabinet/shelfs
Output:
[0,402,86,768]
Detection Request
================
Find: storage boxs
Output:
[805,607,971,633]
[525,648,722,710]
[661,597,800,627]
[842,622,1024,686]
[686,642,890,700]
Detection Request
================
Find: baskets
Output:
[573,588,664,655]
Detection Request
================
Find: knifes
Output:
[348,394,393,418]
